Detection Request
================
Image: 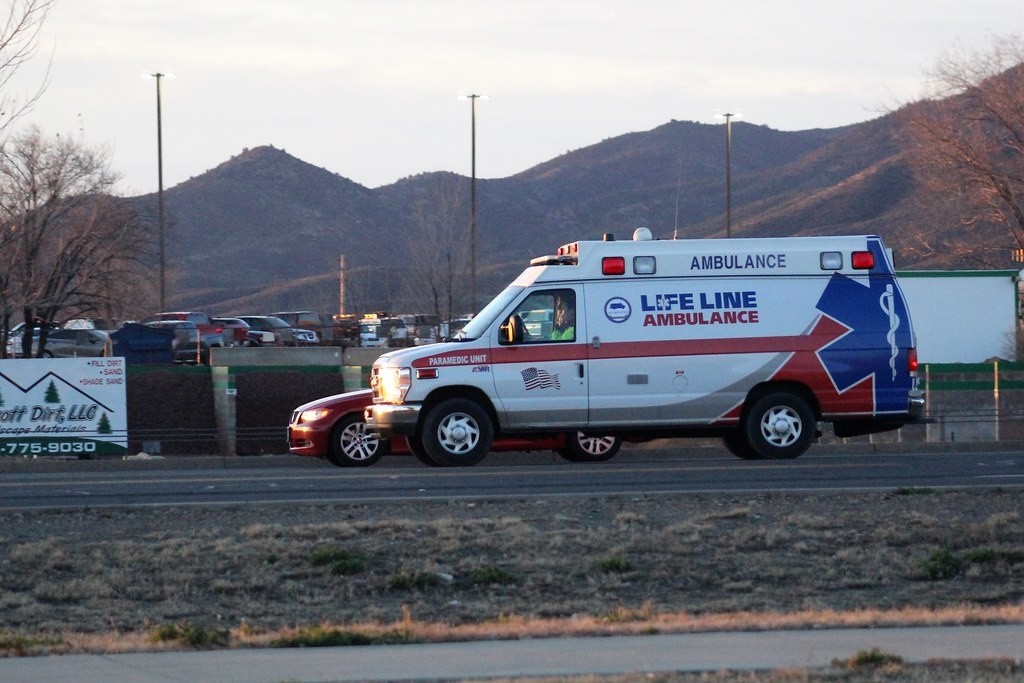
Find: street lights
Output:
[467,94,480,312]
[724,113,733,238]
[152,73,166,312]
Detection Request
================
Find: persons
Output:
[551,295,575,341]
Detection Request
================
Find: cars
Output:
[288,389,622,467]
[0,310,555,365]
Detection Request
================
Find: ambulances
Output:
[365,235,926,468]
